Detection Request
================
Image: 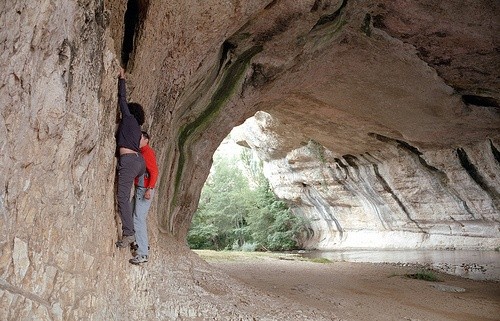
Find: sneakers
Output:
[133,245,151,251]
[129,255,148,263]
[116,236,135,247]
